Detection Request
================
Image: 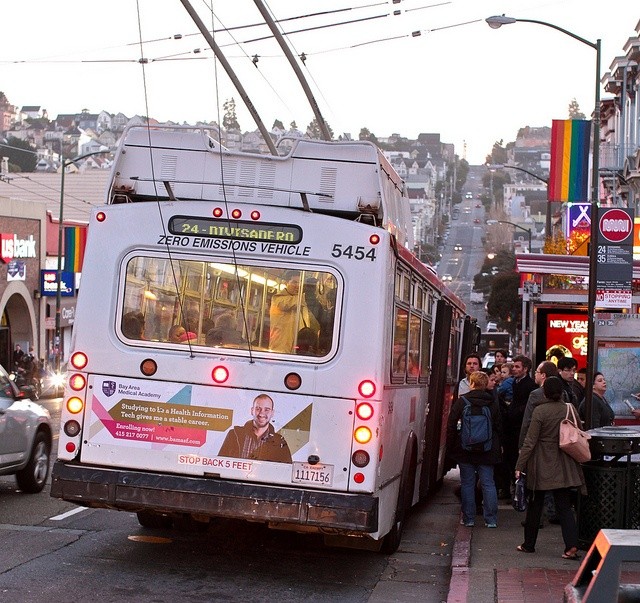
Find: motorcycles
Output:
[8,368,43,402]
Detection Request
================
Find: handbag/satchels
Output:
[515,472,526,511]
[559,403,591,462]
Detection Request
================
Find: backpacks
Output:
[461,395,493,453]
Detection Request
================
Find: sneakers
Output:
[457,517,474,526]
[485,522,496,527]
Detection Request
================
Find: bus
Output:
[54,125,482,557]
[469,283,484,304]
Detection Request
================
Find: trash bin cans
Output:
[574,426,639,551]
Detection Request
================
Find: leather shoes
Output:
[498,493,511,499]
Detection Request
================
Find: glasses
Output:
[563,368,575,371]
[535,370,541,373]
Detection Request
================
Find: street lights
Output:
[487,165,552,241]
[486,15,601,433]
[55,146,110,369]
[486,220,531,253]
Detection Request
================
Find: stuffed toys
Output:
[456,419,462,430]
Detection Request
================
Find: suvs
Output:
[0,370,52,493]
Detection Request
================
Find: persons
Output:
[515,376,592,560]
[491,351,507,370]
[518,360,559,456]
[557,357,578,403]
[479,368,495,390]
[497,363,515,405]
[492,364,504,385]
[13,344,24,362]
[577,368,587,388]
[393,352,413,377]
[445,371,504,528]
[578,372,615,432]
[506,354,540,425]
[122,268,337,356]
[217,394,293,464]
[451,353,482,413]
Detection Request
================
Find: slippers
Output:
[517,545,534,552]
[562,551,581,559]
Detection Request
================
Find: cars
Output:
[454,242,463,251]
[465,191,473,199]
[442,274,453,282]
